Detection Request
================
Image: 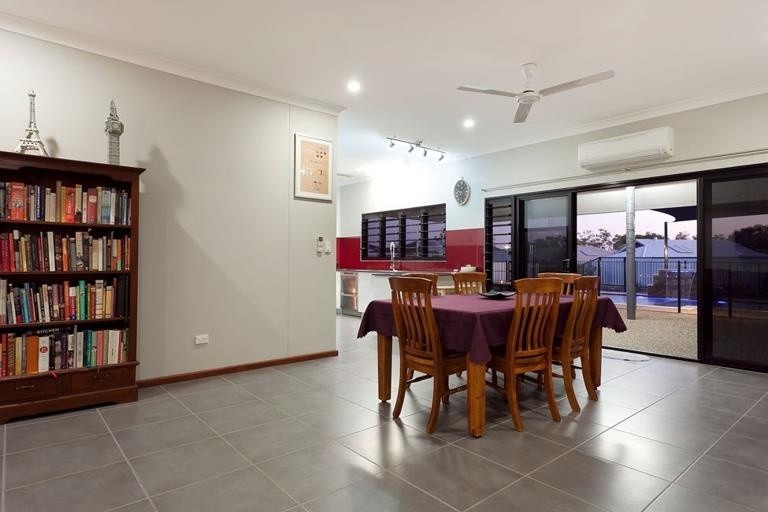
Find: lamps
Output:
[386,136,445,162]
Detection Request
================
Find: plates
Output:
[474,288,517,299]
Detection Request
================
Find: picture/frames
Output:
[293,134,333,204]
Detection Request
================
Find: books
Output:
[0,277,131,325]
[0,181,131,226]
[0,230,130,271]
[0,325,130,379]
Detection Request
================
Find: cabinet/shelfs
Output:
[335,272,340,309]
[370,274,394,301]
[357,273,370,313]
[0,153,146,425]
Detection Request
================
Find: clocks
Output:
[453,179,470,206]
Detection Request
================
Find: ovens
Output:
[340,272,361,317]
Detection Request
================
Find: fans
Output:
[457,62,616,123]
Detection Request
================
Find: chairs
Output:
[388,272,598,440]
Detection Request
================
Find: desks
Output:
[355,291,627,406]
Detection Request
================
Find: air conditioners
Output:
[578,126,675,172]
